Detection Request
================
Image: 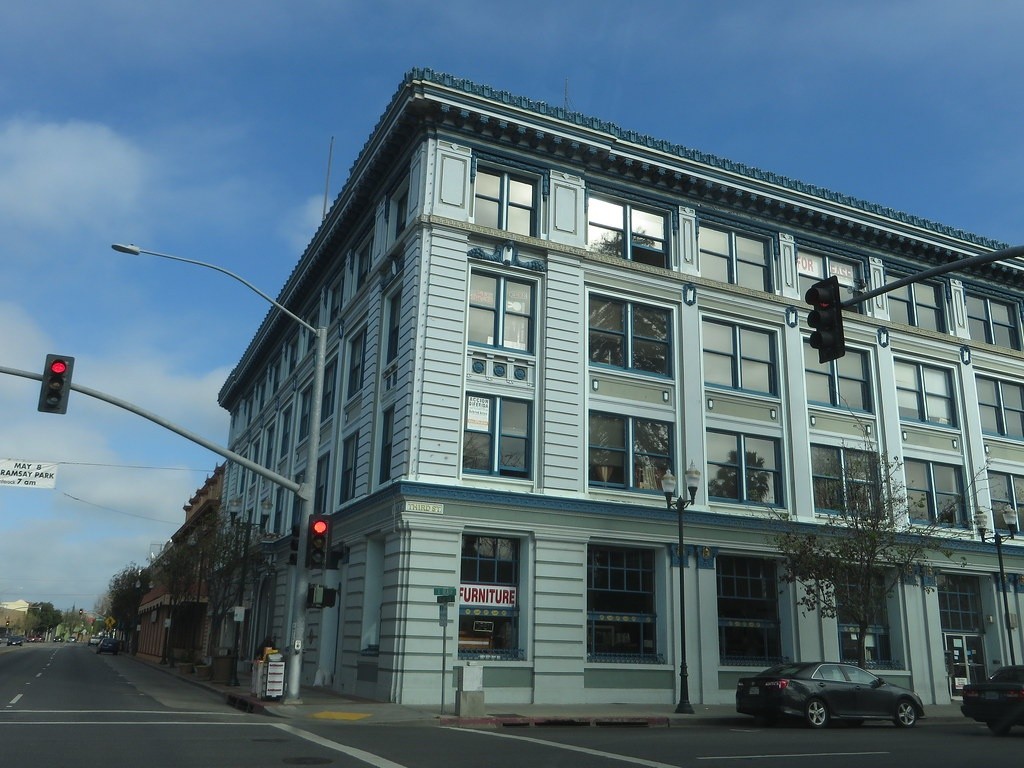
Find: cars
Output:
[7,635,23,646]
[960,664,1024,735]
[26,633,45,643]
[96,637,120,655]
[87,635,102,647]
[66,637,78,643]
[736,661,925,729]
[53,636,63,643]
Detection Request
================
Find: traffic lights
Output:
[289,524,301,565]
[79,608,83,615]
[805,276,846,364]
[305,514,330,570]
[6,620,10,626]
[37,354,75,415]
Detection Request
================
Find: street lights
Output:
[974,504,1024,665]
[660,460,702,714]
[111,243,328,706]
